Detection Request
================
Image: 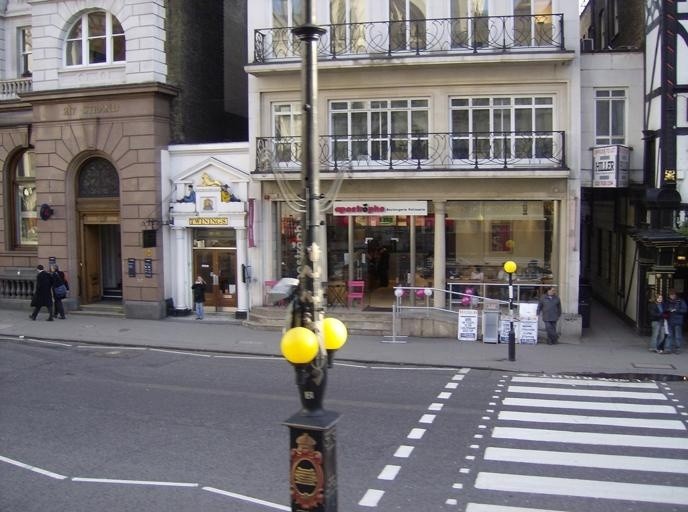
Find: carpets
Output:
[362,304,393,312]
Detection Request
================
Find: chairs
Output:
[346,280,366,311]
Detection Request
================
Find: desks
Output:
[446,277,544,308]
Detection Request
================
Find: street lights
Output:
[504,261,517,361]
[280,317,347,512]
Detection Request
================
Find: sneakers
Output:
[46,315,65,321]
[29,315,35,320]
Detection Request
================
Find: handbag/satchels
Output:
[55,285,67,295]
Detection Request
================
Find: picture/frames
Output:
[489,222,513,255]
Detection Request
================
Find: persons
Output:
[221,185,240,202]
[537,287,562,345]
[191,275,208,319]
[469,260,552,300]
[667,288,687,355]
[375,246,390,287]
[29,264,53,321]
[176,184,197,203]
[649,295,669,356]
[49,264,69,319]
